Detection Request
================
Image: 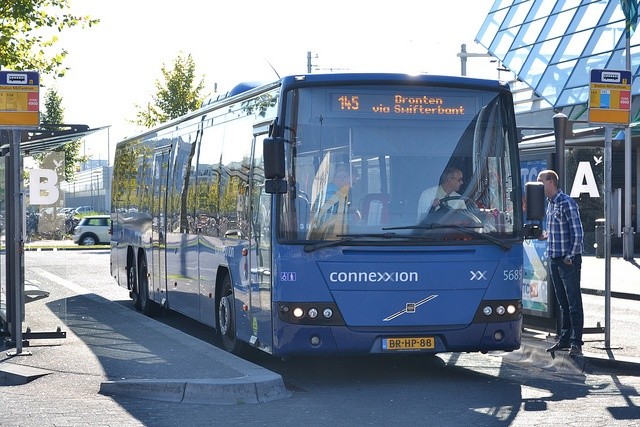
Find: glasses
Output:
[446,176,463,182]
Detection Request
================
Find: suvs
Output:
[74,216,113,245]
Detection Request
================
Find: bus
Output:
[112,73,544,363]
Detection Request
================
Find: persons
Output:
[315,163,358,228]
[417,167,468,222]
[537,169,584,356]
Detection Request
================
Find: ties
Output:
[444,195,449,207]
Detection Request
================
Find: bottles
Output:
[495,210,505,232]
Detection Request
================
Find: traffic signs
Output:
[1,71,40,131]
[590,69,631,128]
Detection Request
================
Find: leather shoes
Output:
[545,339,570,351]
[569,345,581,355]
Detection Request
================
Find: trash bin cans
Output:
[594,218,606,258]
